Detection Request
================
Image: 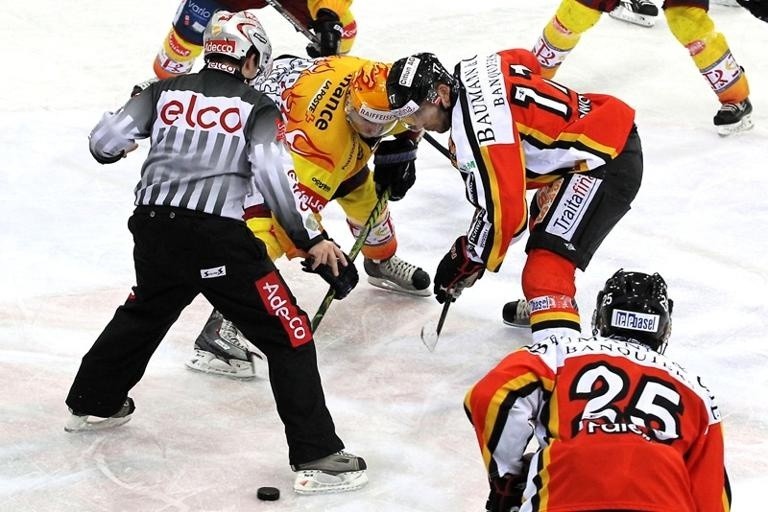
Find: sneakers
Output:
[502,298,580,326]
[111,396,136,418]
[714,98,753,124]
[289,449,367,473]
[631,0,658,17]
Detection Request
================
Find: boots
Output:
[363,253,430,291]
[194,308,254,361]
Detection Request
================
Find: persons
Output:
[187,50,433,366]
[463,265,734,512]
[153,1,362,81]
[60,10,369,475]
[384,45,644,343]
[608,0,660,16]
[531,0,752,126]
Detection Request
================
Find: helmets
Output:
[385,52,466,119]
[591,269,675,353]
[202,8,273,73]
[344,61,400,139]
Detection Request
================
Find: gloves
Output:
[305,8,345,58]
[434,235,487,304]
[301,244,358,299]
[373,136,416,202]
[485,454,530,511]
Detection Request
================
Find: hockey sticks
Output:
[420,300,451,352]
[609,5,653,27]
